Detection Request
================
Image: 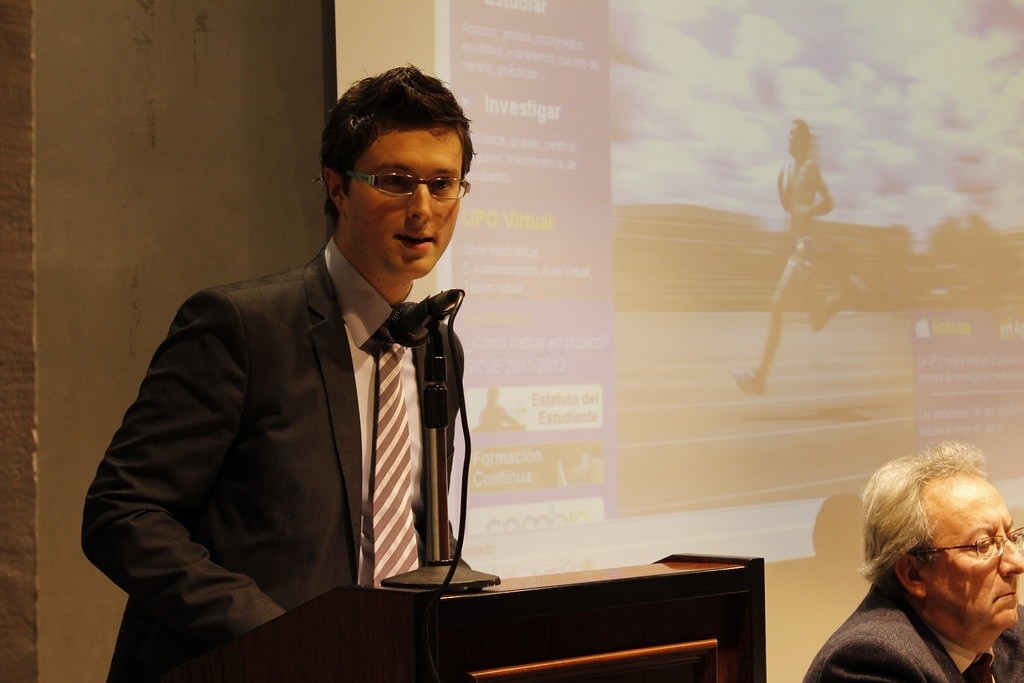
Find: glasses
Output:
[352,172,470,200]
[929,527,1024,559]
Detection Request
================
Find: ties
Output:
[373,344,419,591]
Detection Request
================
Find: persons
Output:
[82,66,473,683]
[733,117,880,406]
[799,442,1024,683]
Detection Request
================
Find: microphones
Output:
[388,288,461,347]
